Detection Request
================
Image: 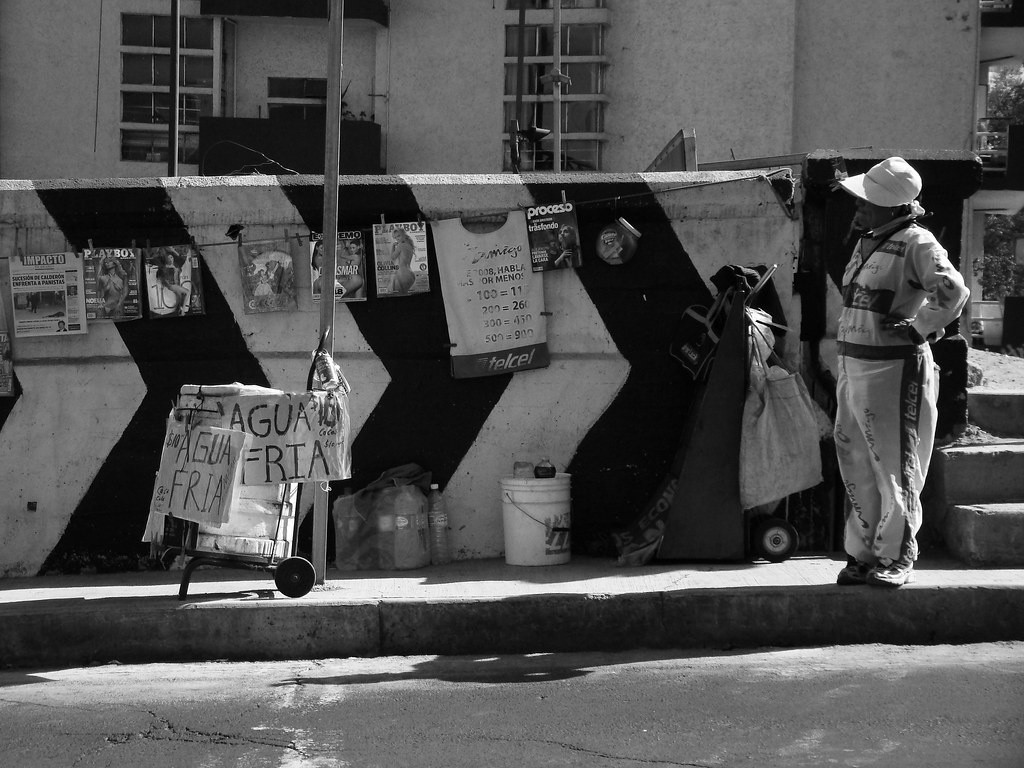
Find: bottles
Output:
[430,484,449,565]
[534,456,556,479]
[334,484,429,570]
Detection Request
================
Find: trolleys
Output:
[611,262,800,567]
[158,322,333,598]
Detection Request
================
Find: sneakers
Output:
[866,558,912,588]
[835,556,868,586]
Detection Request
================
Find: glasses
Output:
[841,268,863,305]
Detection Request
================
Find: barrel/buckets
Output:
[971,301,1005,346]
[499,472,572,565]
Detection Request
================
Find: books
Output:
[309,230,367,303]
[82,248,143,322]
[143,243,206,319]
[525,200,582,272]
[238,241,299,314]
[373,221,431,298]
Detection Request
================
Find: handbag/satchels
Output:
[670,286,733,380]
[740,334,825,513]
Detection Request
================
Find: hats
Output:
[839,157,926,217]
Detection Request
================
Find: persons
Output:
[97,256,129,317]
[158,253,188,316]
[390,228,416,294]
[548,225,580,269]
[56,321,67,332]
[68,286,77,296]
[343,240,365,298]
[311,240,323,294]
[832,156,970,586]
